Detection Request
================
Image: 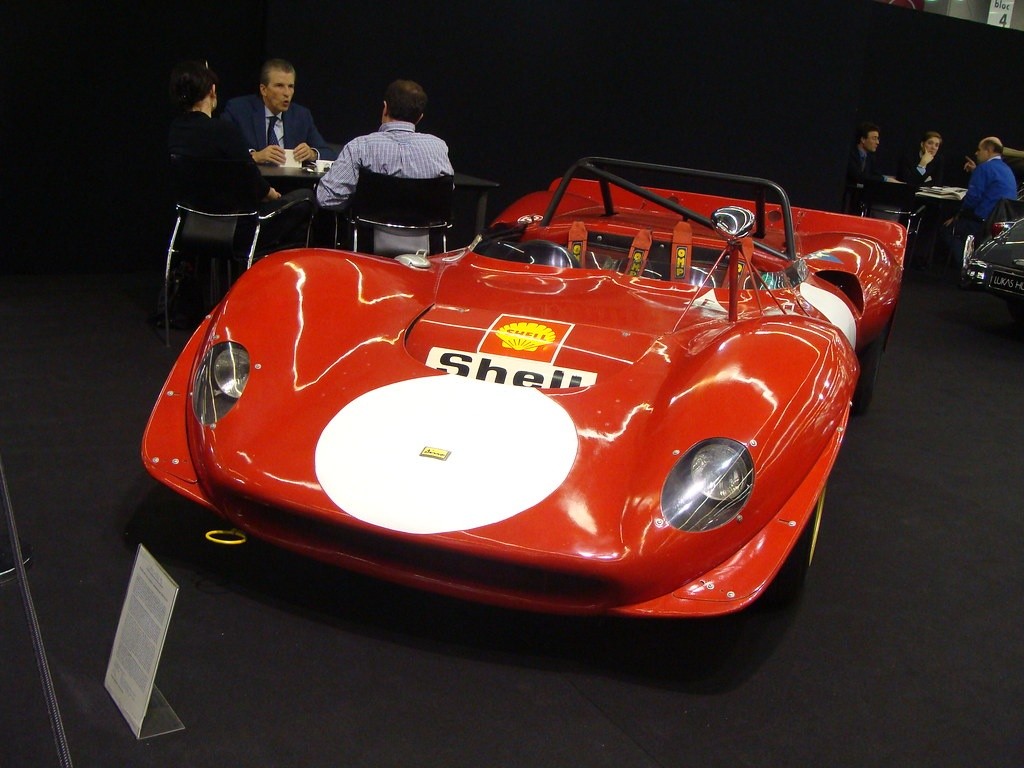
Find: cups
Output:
[316,160,334,172]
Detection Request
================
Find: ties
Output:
[268,116,279,144]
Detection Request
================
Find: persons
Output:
[843,122,1018,271]
[169,59,455,259]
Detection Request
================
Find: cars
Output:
[957,216,1024,324]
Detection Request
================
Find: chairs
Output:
[164,153,316,354]
[943,198,1024,279]
[335,166,454,258]
[857,182,926,273]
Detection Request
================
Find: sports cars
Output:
[145,161,910,623]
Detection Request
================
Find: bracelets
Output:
[918,166,926,169]
[313,149,318,159]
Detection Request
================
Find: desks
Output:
[254,163,500,238]
[913,184,969,275]
